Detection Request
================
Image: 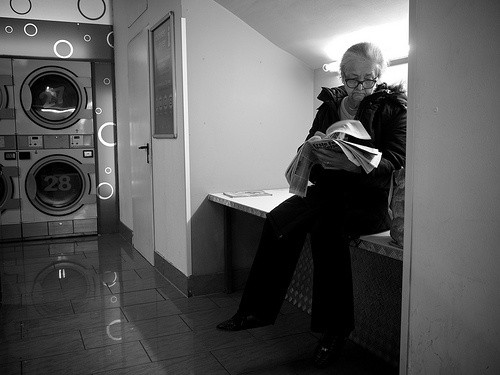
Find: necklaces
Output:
[345,98,358,111]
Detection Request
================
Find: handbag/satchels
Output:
[388,163,406,248]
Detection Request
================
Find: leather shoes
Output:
[312,333,345,370]
[216,313,270,331]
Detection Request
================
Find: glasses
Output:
[342,70,379,89]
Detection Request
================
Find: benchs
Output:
[209,187,403,363]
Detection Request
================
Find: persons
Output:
[216,45,407,375]
